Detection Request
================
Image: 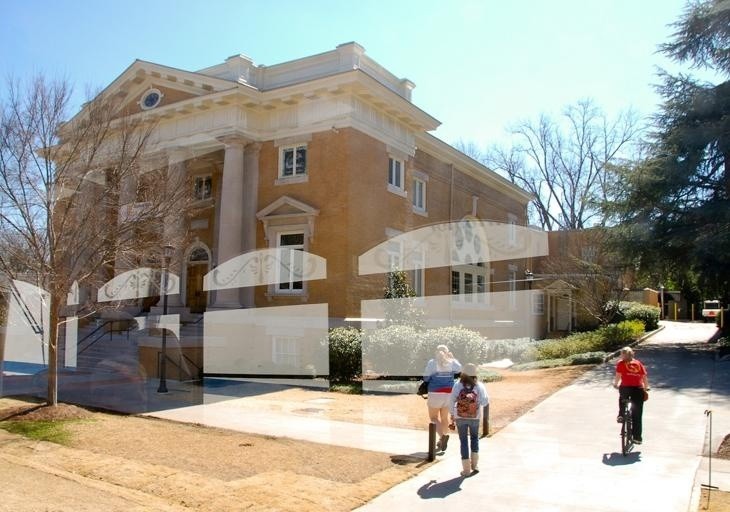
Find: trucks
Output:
[701,300,722,320]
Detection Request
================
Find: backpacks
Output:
[457,384,477,418]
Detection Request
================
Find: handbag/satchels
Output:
[417,382,429,395]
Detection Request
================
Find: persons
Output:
[446,362,490,478]
[612,345,649,445]
[422,342,462,453]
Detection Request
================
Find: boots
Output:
[471,453,479,472]
[461,460,470,477]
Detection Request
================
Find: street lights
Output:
[527,272,535,290]
[157,244,174,394]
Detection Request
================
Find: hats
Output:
[463,364,479,376]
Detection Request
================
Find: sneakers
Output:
[440,435,449,451]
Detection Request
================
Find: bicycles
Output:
[614,386,652,456]
